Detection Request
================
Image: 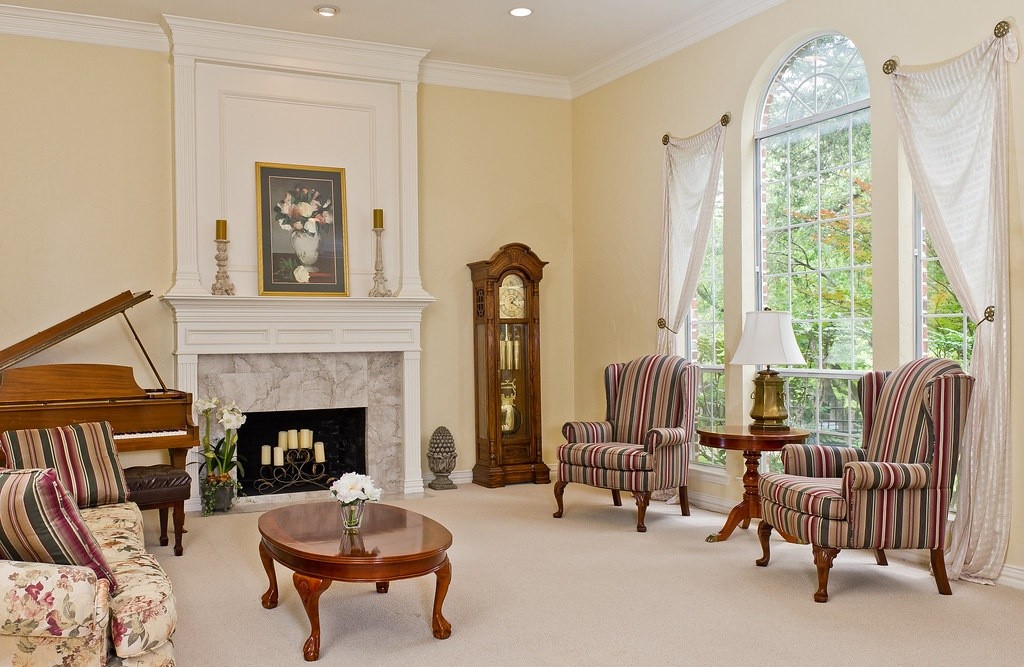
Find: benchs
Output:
[119,464,191,558]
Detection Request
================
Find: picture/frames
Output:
[254,161,350,299]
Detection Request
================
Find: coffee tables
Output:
[257,501,452,662]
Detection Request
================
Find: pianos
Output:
[0,289,201,534]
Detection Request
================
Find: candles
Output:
[261,445,271,465]
[372,208,384,229]
[274,446,284,466]
[216,219,228,240]
[277,428,313,450]
[313,442,325,463]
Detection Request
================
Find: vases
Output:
[199,483,230,510]
[337,500,365,529]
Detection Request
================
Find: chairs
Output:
[552,355,701,534]
[753,357,978,602]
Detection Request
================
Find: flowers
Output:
[179,396,255,517]
[329,471,382,527]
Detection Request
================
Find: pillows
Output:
[0,420,131,510]
[0,465,118,596]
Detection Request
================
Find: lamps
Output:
[729,307,809,434]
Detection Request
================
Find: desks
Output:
[694,424,810,544]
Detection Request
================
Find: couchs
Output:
[0,505,179,667]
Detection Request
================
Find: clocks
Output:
[465,241,551,489]
